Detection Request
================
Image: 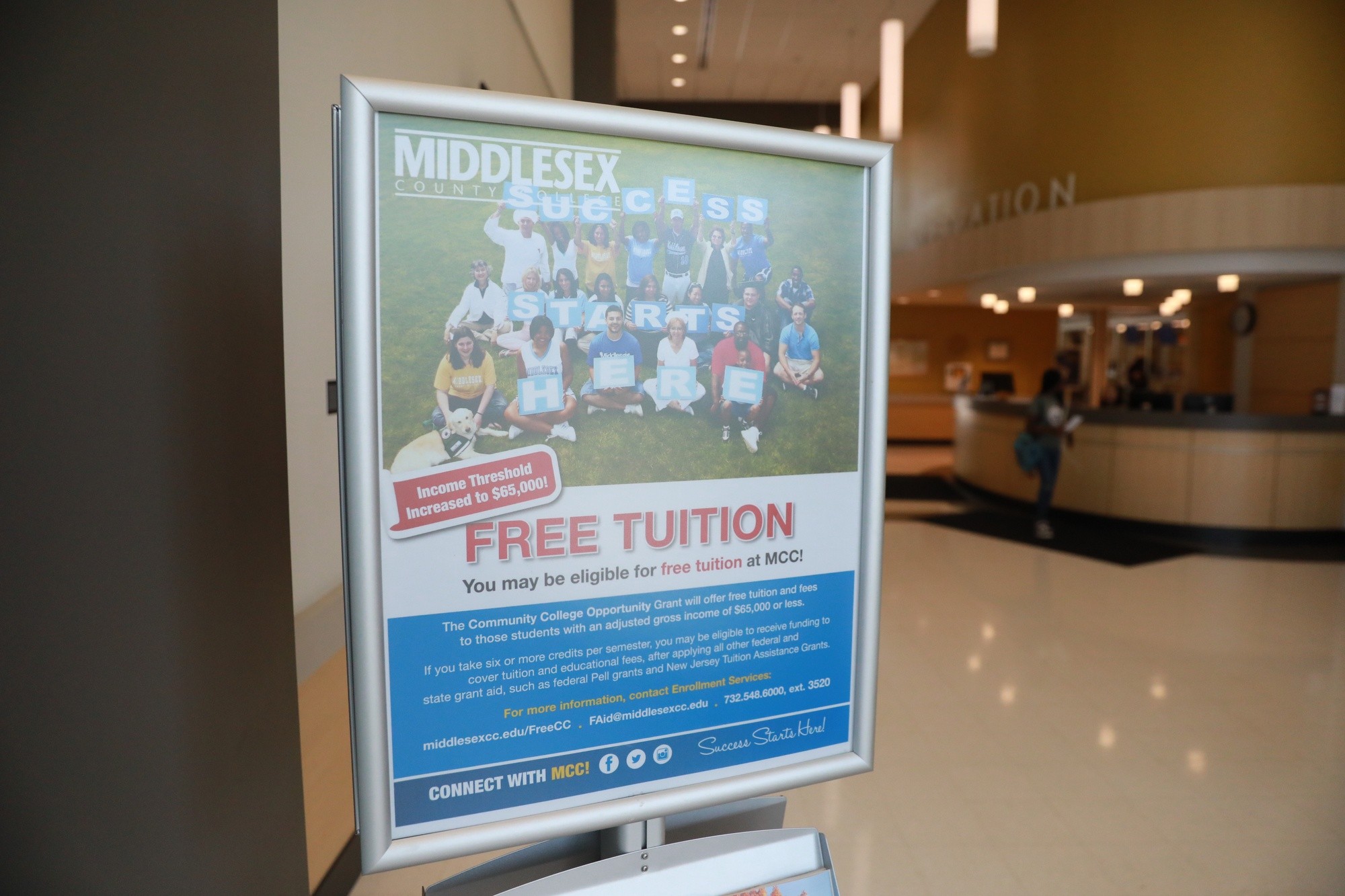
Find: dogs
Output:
[390,408,493,475]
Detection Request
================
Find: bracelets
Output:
[476,411,485,416]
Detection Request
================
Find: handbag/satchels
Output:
[1015,425,1052,475]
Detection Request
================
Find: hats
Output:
[671,209,685,219]
[513,209,538,226]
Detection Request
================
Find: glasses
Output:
[688,282,703,292]
[712,234,722,239]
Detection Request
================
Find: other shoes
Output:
[1034,520,1055,538]
[738,417,752,430]
[723,427,731,441]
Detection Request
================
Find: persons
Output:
[482,198,774,357]
[1029,367,1083,544]
[775,266,816,329]
[719,349,764,441]
[710,283,773,374]
[579,305,645,417]
[443,259,508,345]
[503,315,577,442]
[432,326,506,431]
[643,317,707,416]
[774,303,824,399]
[722,283,774,374]
[709,321,778,453]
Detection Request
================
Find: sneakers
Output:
[626,404,643,418]
[741,428,761,456]
[587,406,606,416]
[804,386,818,399]
[656,405,658,412]
[685,408,696,416]
[509,425,523,440]
[782,383,793,391]
[551,421,576,443]
